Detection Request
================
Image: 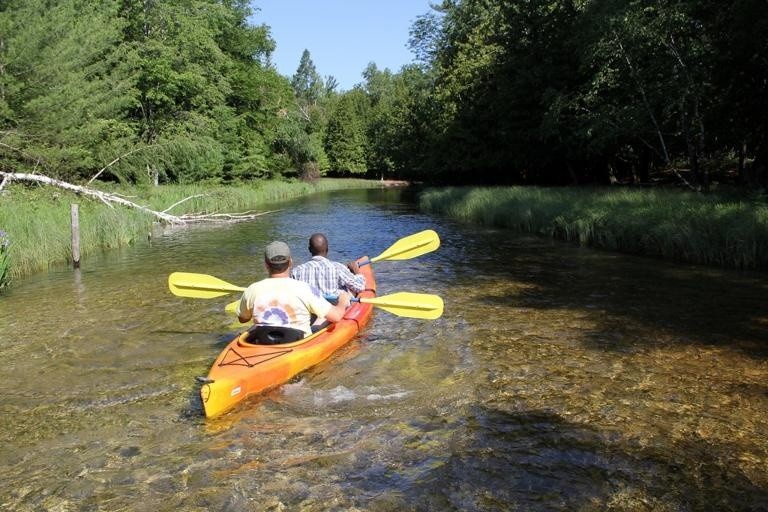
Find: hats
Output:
[265,241,290,264]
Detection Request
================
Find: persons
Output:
[235,239,352,341]
[289,233,366,312]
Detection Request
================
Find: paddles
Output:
[167,273,443,319]
[224,228,441,311]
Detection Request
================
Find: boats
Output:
[198,256,377,420]
